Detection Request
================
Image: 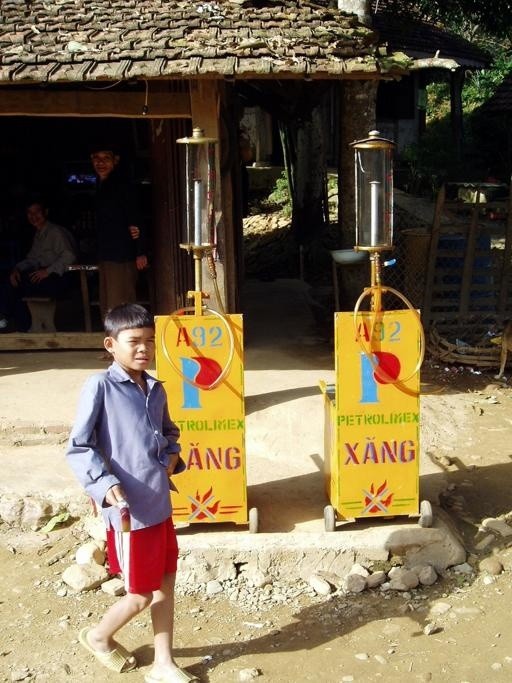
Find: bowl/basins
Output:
[330,248,368,265]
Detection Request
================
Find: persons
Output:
[85,137,150,360]
[65,298,205,682]
[0,193,77,332]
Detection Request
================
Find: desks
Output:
[66,263,156,332]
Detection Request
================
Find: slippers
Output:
[80,626,138,673]
[142,664,201,683]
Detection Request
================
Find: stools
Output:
[23,296,58,332]
